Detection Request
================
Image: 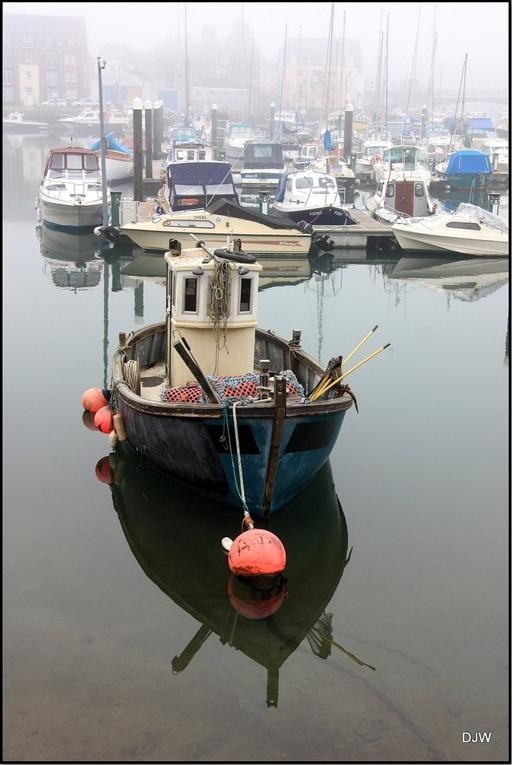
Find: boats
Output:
[108,239,358,523]
[3,3,511,299]
[108,435,354,711]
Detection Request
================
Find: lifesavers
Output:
[215,248,256,264]
